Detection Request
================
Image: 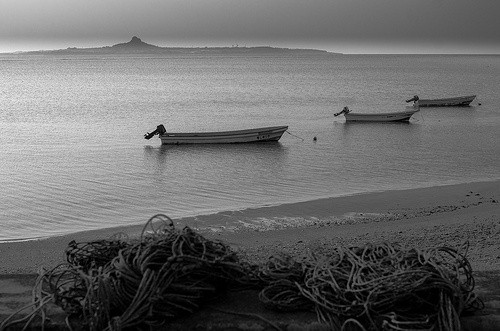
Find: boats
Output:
[406,94,477,107]
[334,107,414,122]
[144,124,288,144]
